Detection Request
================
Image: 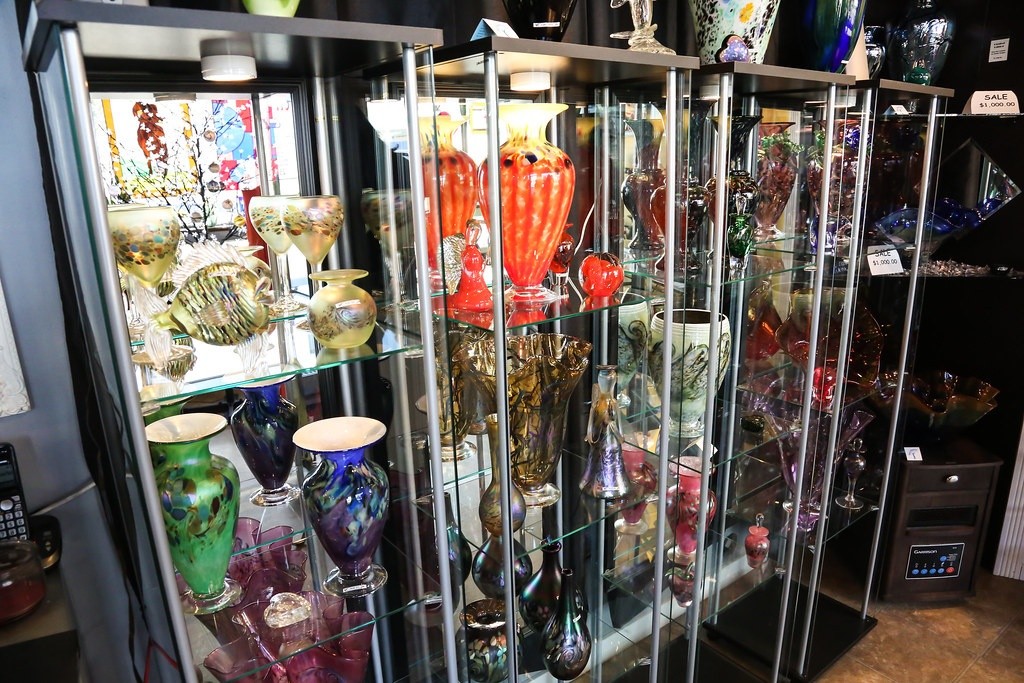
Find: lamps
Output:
[200,56,257,82]
[836,96,856,108]
[700,85,720,100]
[510,72,550,91]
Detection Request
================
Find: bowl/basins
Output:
[173,513,375,682]
[875,365,1000,434]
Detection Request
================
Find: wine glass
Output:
[104,195,346,363]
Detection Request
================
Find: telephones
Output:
[0,441,63,573]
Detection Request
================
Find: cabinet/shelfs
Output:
[21,0,1024,683]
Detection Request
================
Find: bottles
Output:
[309,268,376,349]
[443,490,590,680]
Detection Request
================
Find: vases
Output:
[496,101,575,304]
[805,117,861,242]
[144,411,243,617]
[229,375,301,507]
[758,121,797,241]
[706,115,765,269]
[615,434,656,537]
[621,119,666,252]
[668,457,717,567]
[649,97,720,274]
[292,415,388,599]
[413,113,477,294]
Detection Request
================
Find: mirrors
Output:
[365,95,563,670]
[737,105,804,501]
[80,84,375,683]
[613,95,703,597]
